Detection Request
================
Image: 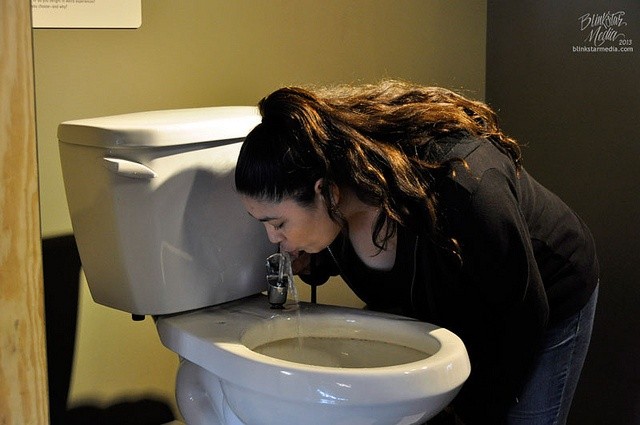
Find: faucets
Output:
[263,249,295,309]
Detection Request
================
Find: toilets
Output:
[57,105,473,425]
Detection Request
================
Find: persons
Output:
[235,76,603,424]
